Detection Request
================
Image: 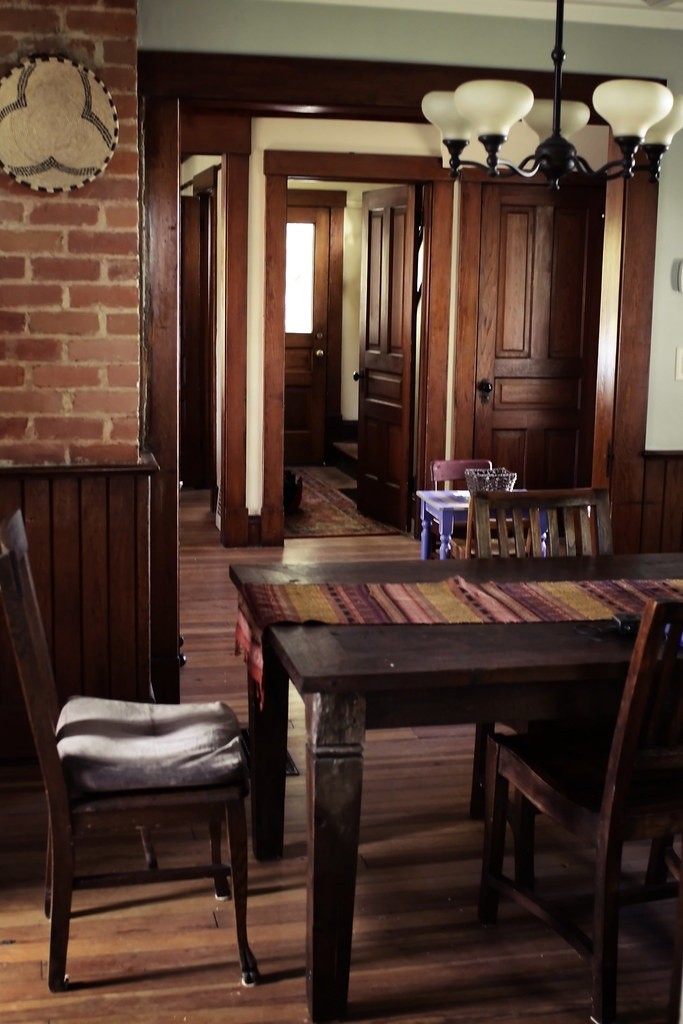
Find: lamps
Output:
[420,0,683,192]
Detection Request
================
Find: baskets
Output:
[463,466,518,497]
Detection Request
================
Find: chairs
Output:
[484,596,683,1023]
[0,510,267,994]
[428,459,492,490]
[467,485,614,555]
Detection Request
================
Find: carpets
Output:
[281,468,403,539]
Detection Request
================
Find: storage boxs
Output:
[464,467,518,492]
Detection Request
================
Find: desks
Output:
[224,554,683,1023]
[416,487,552,560]
